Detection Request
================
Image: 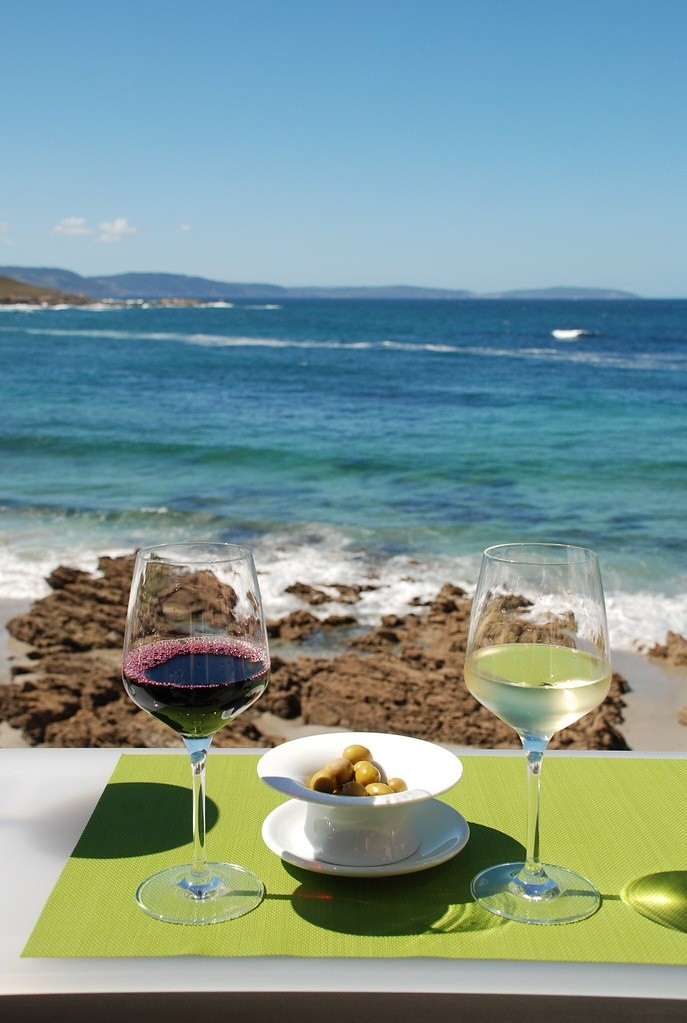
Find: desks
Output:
[0,746,687,1023]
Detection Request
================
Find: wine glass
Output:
[119,543,265,927]
[463,542,613,925]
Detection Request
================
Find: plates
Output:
[257,798,478,877]
